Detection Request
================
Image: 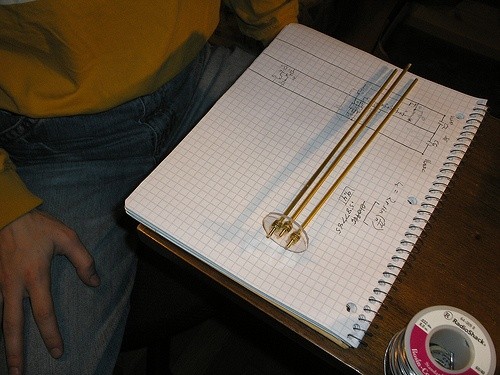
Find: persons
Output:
[0,0,301,374]
